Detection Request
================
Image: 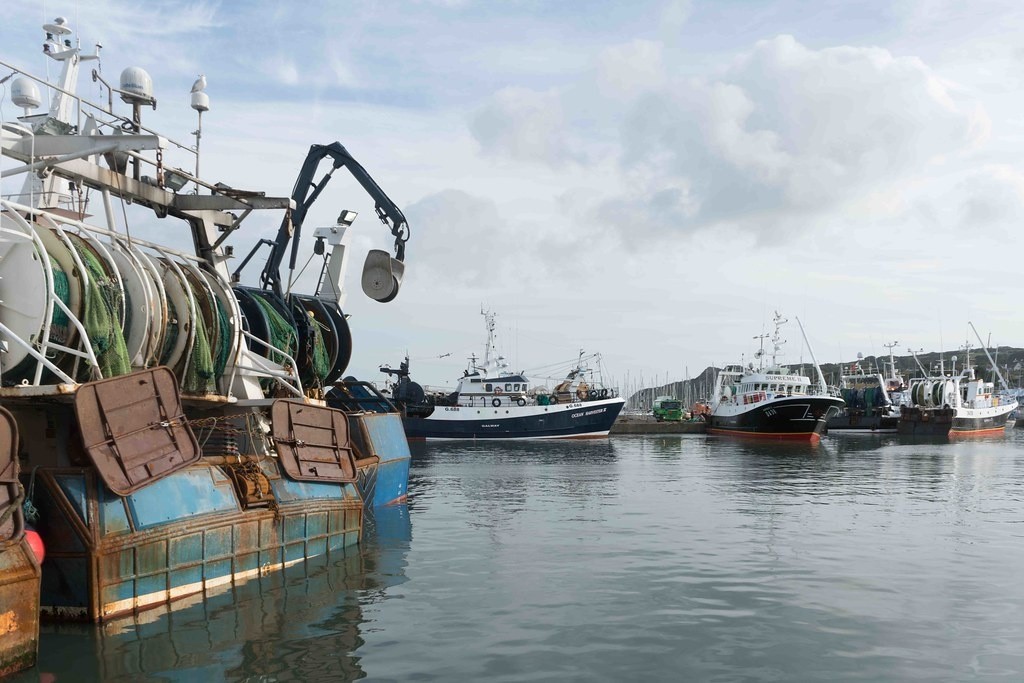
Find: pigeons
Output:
[190,73,207,93]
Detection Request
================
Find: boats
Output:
[807,321,1024,437]
[699,310,845,441]
[387,301,626,441]
[1,14,413,678]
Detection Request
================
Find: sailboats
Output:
[617,363,718,415]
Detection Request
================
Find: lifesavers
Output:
[601,389,607,399]
[590,390,598,399]
[548,395,558,404]
[494,386,502,395]
[492,398,501,407]
[517,398,526,406]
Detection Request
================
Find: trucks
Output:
[654,396,685,423]
